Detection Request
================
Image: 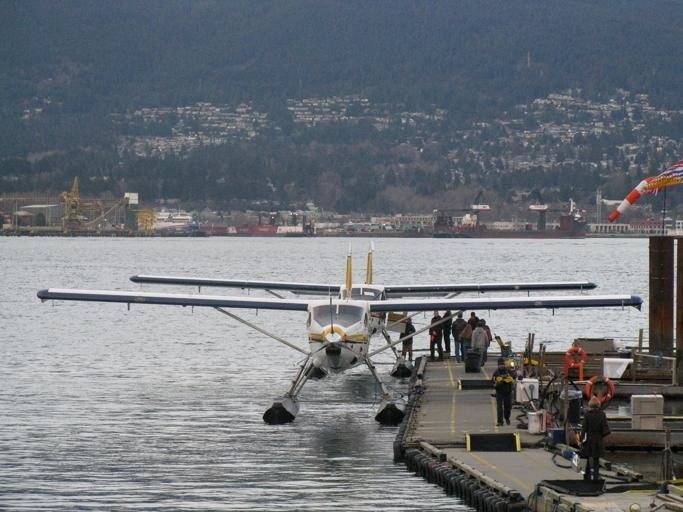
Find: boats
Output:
[429,197,592,238]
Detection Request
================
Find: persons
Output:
[579,395,611,481]
[399,318,416,363]
[493,358,515,427]
[429,311,493,368]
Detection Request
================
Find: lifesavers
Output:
[566,348,587,367]
[586,375,616,403]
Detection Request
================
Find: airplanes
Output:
[37,240,643,424]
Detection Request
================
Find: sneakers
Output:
[495,418,511,427]
[407,355,472,364]
